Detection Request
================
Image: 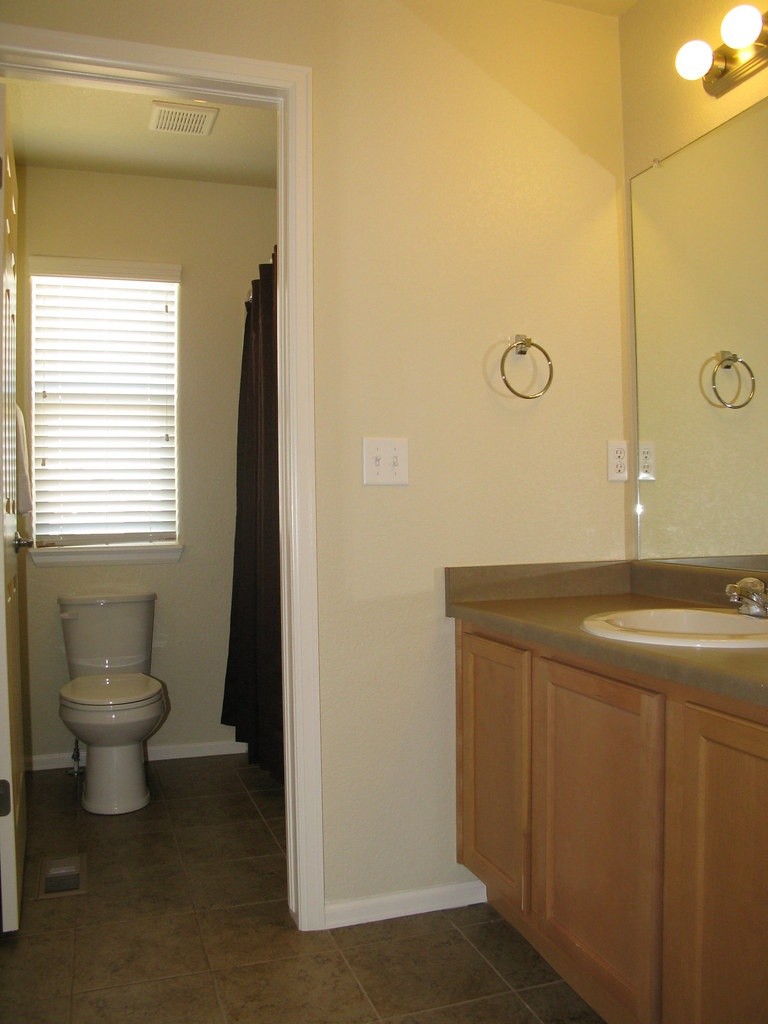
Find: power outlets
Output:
[637,441,657,481]
[607,439,629,481]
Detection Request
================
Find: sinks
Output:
[577,606,768,649]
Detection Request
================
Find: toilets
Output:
[56,591,168,816]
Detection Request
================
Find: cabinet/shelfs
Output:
[456,619,768,1024]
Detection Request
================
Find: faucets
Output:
[725,582,768,619]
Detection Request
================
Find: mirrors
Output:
[630,98,768,575]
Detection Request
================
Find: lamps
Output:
[674,3,768,99]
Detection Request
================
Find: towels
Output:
[15,402,34,518]
